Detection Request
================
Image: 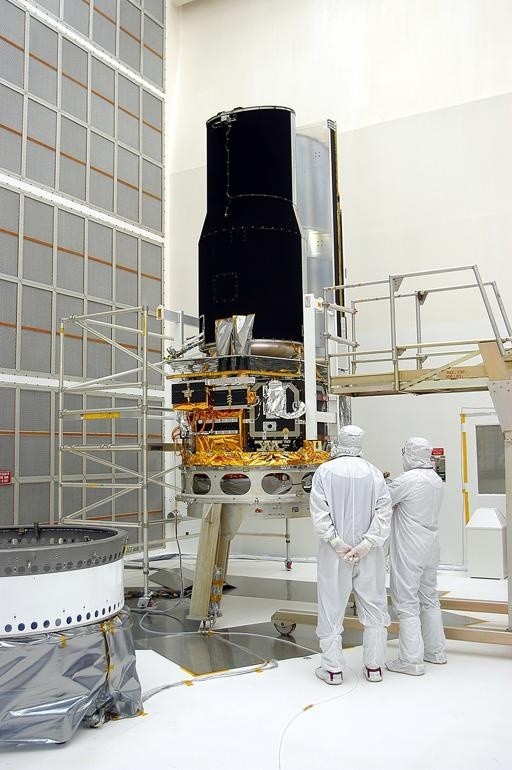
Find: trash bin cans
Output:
[466,507,507,580]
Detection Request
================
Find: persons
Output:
[385,438,447,675]
[309,425,392,685]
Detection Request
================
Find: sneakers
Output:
[363,664,383,682]
[385,652,447,676]
[315,666,344,685]
